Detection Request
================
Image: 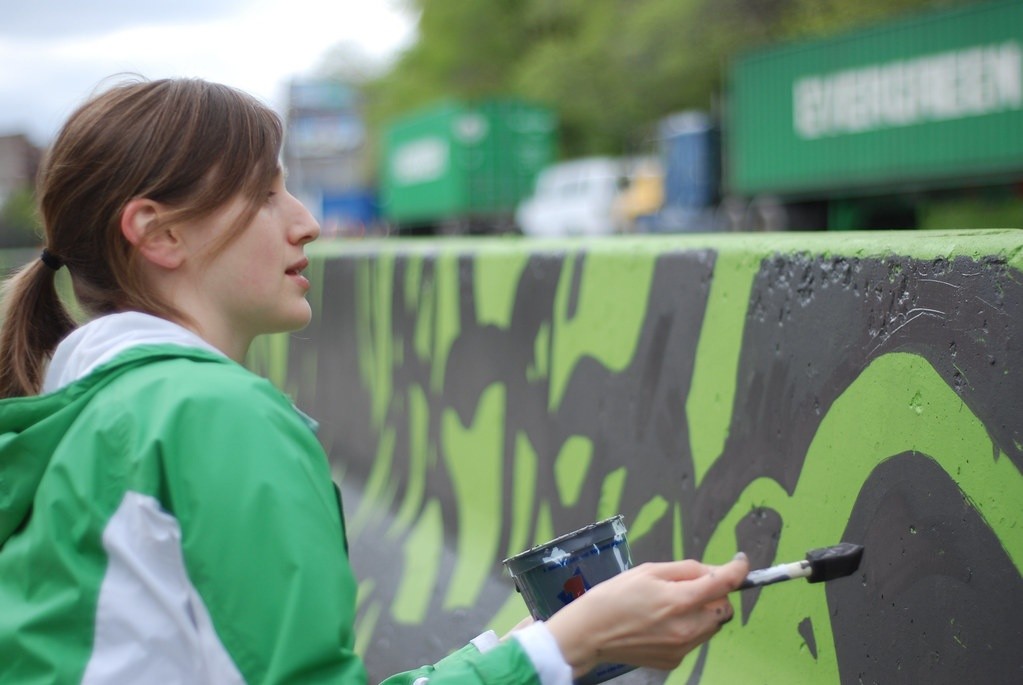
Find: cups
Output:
[502,515,641,685]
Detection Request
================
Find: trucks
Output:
[380,107,560,238]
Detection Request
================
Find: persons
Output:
[0,79,748,685]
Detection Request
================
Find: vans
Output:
[521,155,658,243]
